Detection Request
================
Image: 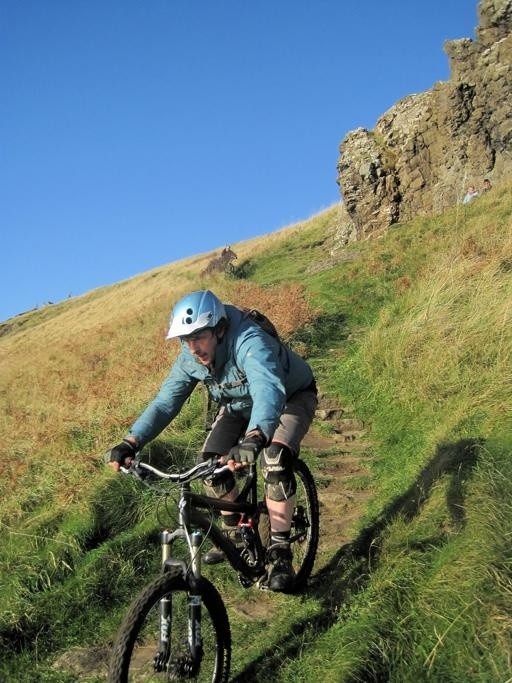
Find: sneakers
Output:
[265,538,293,591]
[202,521,245,565]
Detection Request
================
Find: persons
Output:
[479,178,494,196]
[100,285,322,594]
[219,245,238,270]
[462,184,479,204]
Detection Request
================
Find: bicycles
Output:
[102,447,320,682]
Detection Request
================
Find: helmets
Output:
[164,290,228,340]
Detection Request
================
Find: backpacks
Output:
[239,307,278,339]
